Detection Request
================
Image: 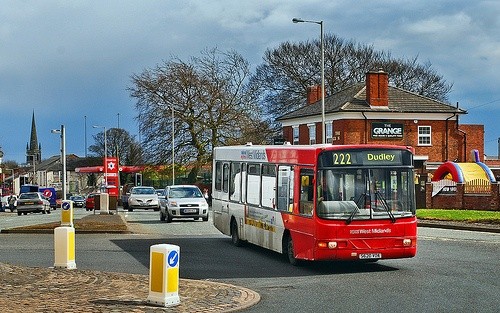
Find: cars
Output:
[16,191,51,216]
[69,196,86,208]
[86,193,101,211]
[126,186,160,212]
[157,185,209,223]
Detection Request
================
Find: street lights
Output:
[292,16,326,143]
[158,102,174,185]
[92,125,107,185]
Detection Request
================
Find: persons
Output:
[202,188,211,205]
[190,190,196,197]
[317,178,336,202]
[363,184,377,201]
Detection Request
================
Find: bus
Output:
[212,141,418,266]
